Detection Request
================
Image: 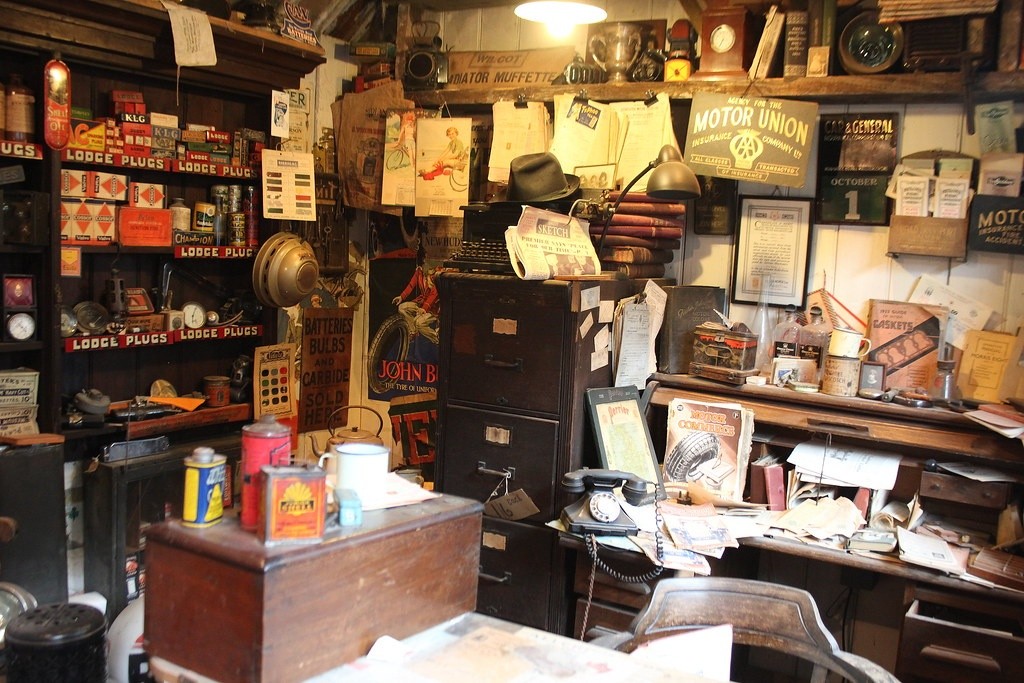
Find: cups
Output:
[317,443,391,512]
[828,326,872,359]
[819,354,862,398]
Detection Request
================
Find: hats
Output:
[487,151,579,203]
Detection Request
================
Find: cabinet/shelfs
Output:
[430,270,679,632]
[562,528,1024,683]
[0,0,330,454]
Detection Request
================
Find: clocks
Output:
[687,4,752,78]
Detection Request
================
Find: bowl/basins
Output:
[838,11,904,74]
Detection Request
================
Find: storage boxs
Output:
[141,488,485,683]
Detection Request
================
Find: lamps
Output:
[598,144,703,259]
[513,0,607,38]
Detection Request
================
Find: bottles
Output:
[203,375,233,406]
[0,72,37,143]
[240,413,292,533]
[768,304,804,363]
[748,273,773,374]
[796,306,829,385]
[996,501,1022,545]
[168,197,191,232]
[931,360,958,401]
[180,447,228,530]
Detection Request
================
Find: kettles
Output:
[308,405,384,469]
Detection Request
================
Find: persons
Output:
[862,368,881,389]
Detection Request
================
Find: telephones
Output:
[559,467,647,536]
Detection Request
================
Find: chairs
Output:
[580,578,898,683]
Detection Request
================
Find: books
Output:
[139,519,151,549]
[897,525,966,576]
[626,501,740,576]
[588,192,686,280]
[846,527,897,553]
[750,454,788,510]
[659,286,726,374]
[964,410,1024,438]
[864,298,950,398]
[748,12,808,78]
